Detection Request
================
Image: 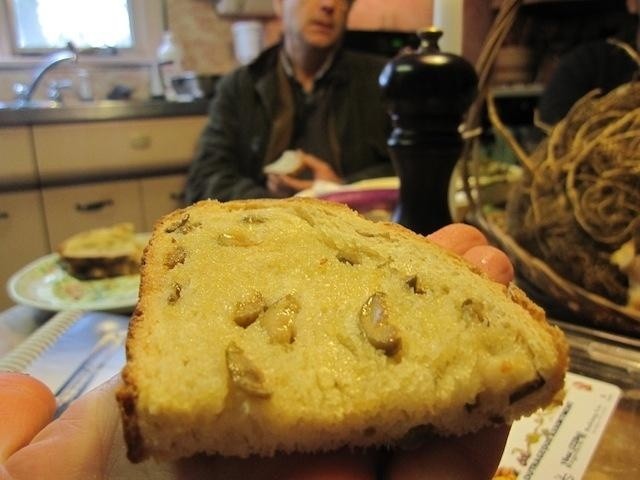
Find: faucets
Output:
[25,52,76,103]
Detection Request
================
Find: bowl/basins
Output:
[172,71,224,101]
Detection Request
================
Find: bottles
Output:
[149,30,186,100]
[77,67,97,101]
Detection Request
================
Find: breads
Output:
[53,220,135,282]
[261,147,307,177]
[113,194,569,468]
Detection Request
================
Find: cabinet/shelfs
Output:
[1,125,51,312]
[33,114,211,253]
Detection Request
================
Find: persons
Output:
[183,0,394,209]
[0,223,515,479]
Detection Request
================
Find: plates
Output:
[4,229,150,316]
